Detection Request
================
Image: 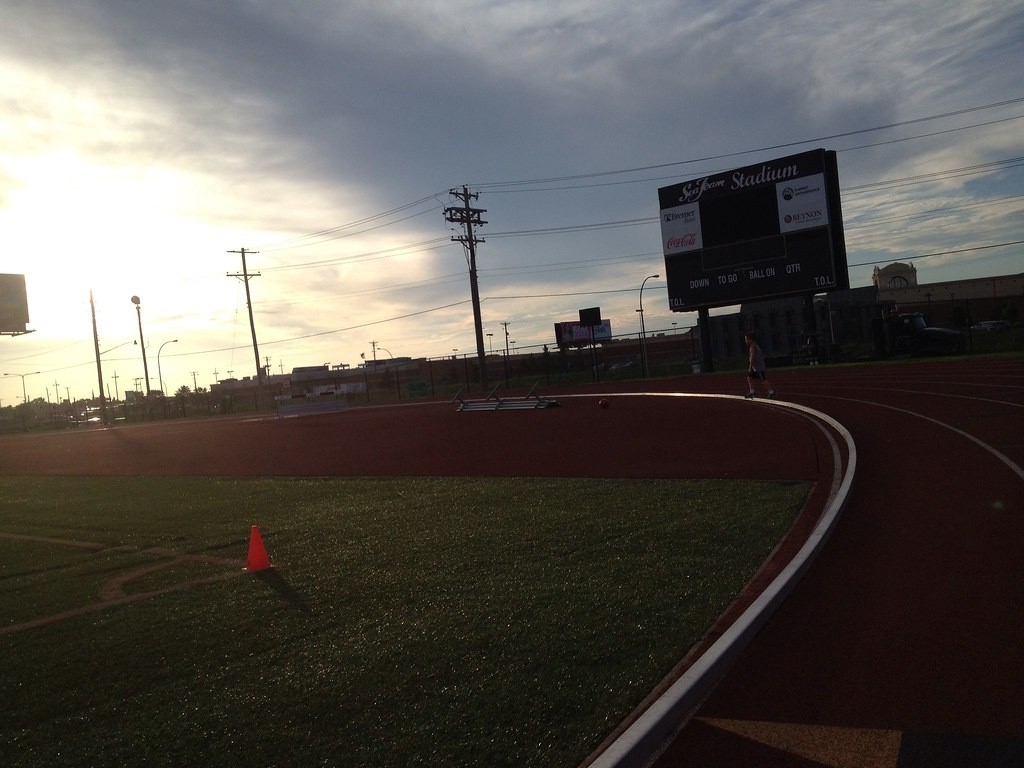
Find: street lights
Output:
[639,275,660,379]
[486,334,494,354]
[4,371,41,404]
[157,340,178,419]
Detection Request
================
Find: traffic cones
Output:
[240,523,277,574]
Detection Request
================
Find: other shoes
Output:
[766,390,778,400]
[744,391,757,398]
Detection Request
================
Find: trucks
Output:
[696,297,969,367]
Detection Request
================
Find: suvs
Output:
[970,320,1009,334]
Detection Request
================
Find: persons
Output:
[744,333,778,401]
